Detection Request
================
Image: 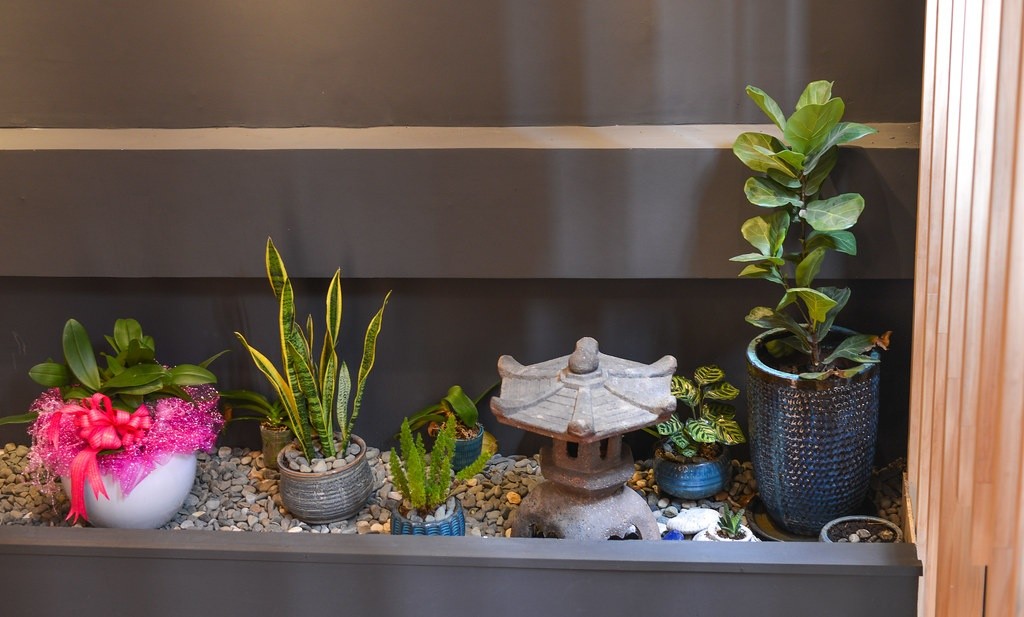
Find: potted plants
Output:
[234,236,392,528]
[731,77,886,539]
[32,316,200,531]
[689,502,762,543]
[392,376,503,476]
[652,364,747,502]
[385,413,498,536]
[216,388,297,468]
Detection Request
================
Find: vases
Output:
[817,514,905,544]
